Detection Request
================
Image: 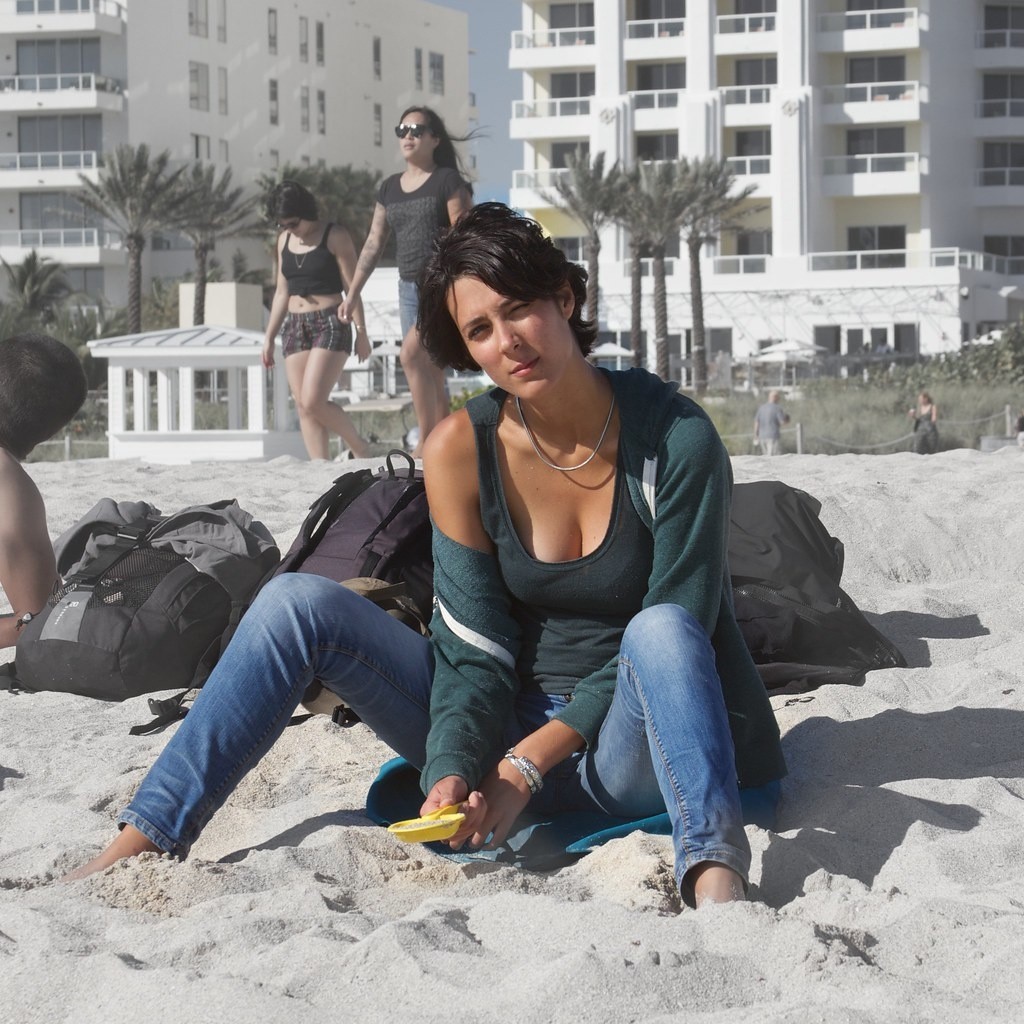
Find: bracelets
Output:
[356,326,365,328]
[505,748,544,794]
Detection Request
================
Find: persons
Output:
[338,105,474,459]
[754,391,789,455]
[909,392,937,455]
[0,336,88,651]
[59,201,783,906]
[261,181,372,459]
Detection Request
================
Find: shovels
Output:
[388,799,469,844]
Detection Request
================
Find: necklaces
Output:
[516,394,615,470]
[295,252,308,268]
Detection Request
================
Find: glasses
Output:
[274,218,301,230]
[395,124,430,138]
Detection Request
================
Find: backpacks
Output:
[16,499,280,704]
[250,447,436,640]
